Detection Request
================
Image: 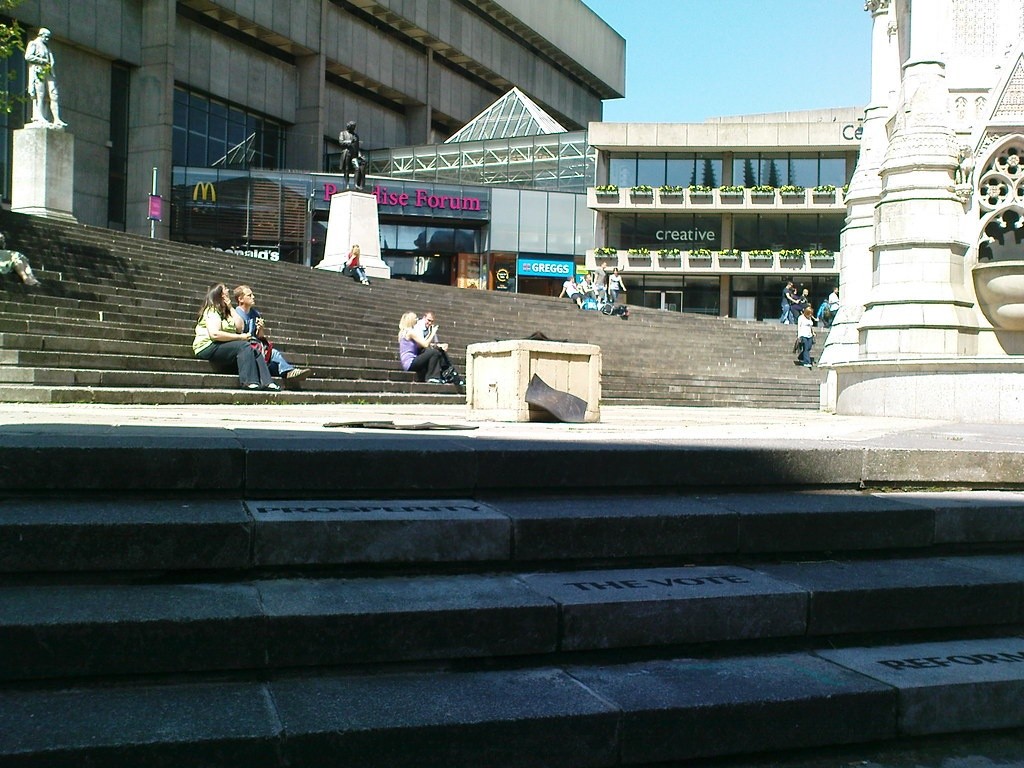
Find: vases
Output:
[720,192,743,196]
[628,255,650,259]
[630,192,652,196]
[718,256,739,259]
[596,191,618,194]
[843,193,847,199]
[811,256,834,260]
[659,192,683,195]
[751,191,774,197]
[596,255,617,258]
[812,192,836,196]
[688,256,711,260]
[658,255,681,260]
[749,256,773,259]
[690,192,712,196]
[780,256,804,260]
[781,192,805,196]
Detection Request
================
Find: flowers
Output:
[658,249,680,255]
[749,249,772,255]
[690,184,711,192]
[719,249,740,256]
[812,184,836,191]
[595,247,616,254]
[779,248,805,256]
[751,184,774,191]
[596,184,617,191]
[659,185,682,191]
[719,185,744,192]
[780,183,805,192]
[631,184,651,191]
[809,249,834,255]
[628,248,649,254]
[842,184,849,191]
[689,248,711,255]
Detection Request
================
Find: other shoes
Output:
[24,279,48,288]
[425,377,444,384]
[262,382,281,391]
[287,368,311,382]
[241,384,259,390]
[804,363,813,367]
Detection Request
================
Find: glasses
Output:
[240,293,254,298]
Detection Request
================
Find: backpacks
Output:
[824,302,832,318]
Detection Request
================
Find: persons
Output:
[192,283,281,392]
[232,285,311,380]
[24,28,68,126]
[339,121,363,192]
[341,245,370,285]
[397,310,464,386]
[798,307,816,368]
[0,230,43,286]
[558,262,626,310]
[780,282,840,326]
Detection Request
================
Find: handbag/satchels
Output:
[441,366,460,384]
[601,305,630,320]
[812,336,816,344]
